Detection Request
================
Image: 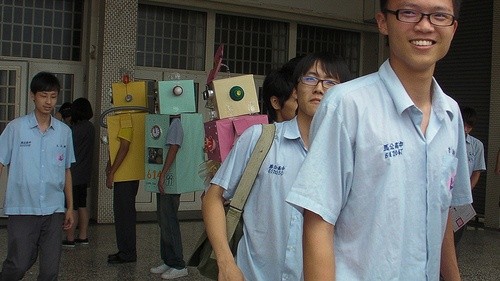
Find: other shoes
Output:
[62,236,75,248]
[75,238,88,245]
[107,252,137,264]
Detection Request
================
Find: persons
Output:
[1,51,355,280]
[286,0,474,281]
[453,108,487,246]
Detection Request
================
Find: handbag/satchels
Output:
[187,123,276,280]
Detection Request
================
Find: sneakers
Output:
[161,267,188,279]
[151,264,170,273]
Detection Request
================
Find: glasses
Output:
[386,7,456,26]
[296,75,340,89]
